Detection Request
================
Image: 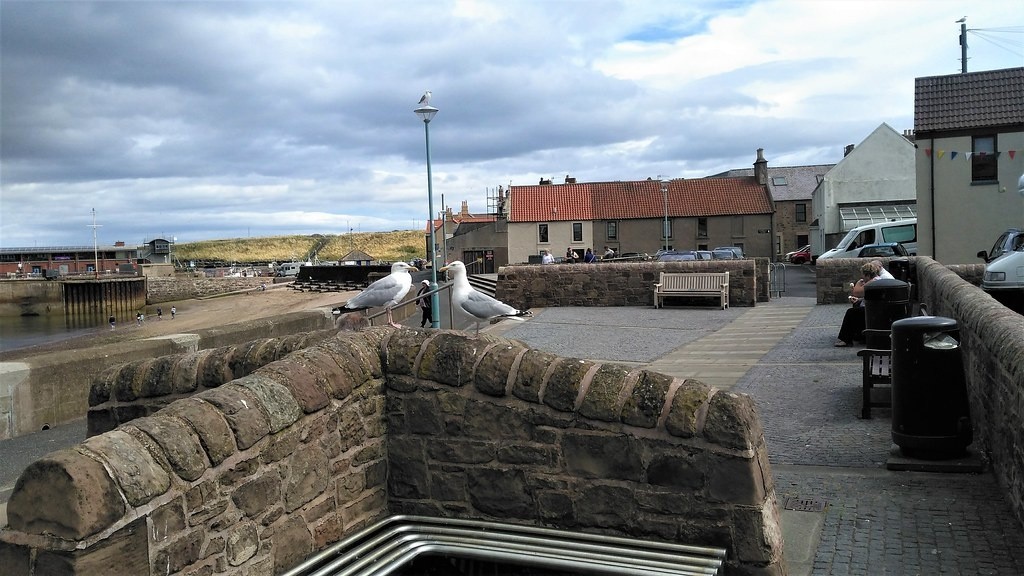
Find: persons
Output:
[541,250,554,264]
[171,306,176,319]
[243,268,247,277]
[253,269,264,277]
[834,260,896,347]
[92,265,96,275]
[109,314,115,332]
[564,247,579,264]
[157,305,163,321]
[416,279,434,328]
[603,245,614,258]
[47,303,51,316]
[262,283,266,291]
[136,310,145,327]
[585,247,597,263]
[415,258,424,271]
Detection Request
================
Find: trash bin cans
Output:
[864,279,910,350]
[886,316,984,473]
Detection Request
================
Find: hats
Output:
[421,280,430,287]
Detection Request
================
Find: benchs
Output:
[906,278,915,319]
[653,271,729,309]
[858,303,929,420]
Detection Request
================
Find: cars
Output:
[621,246,746,261]
[858,242,908,257]
[784,245,810,265]
[977,229,1024,291]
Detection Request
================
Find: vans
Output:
[818,218,917,259]
[275,263,300,277]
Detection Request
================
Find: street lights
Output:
[661,178,671,251]
[414,100,441,329]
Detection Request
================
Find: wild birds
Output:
[435,259,534,338]
[331,260,420,330]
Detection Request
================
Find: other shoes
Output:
[420,326,424,328]
[429,325,433,328]
[834,340,854,347]
